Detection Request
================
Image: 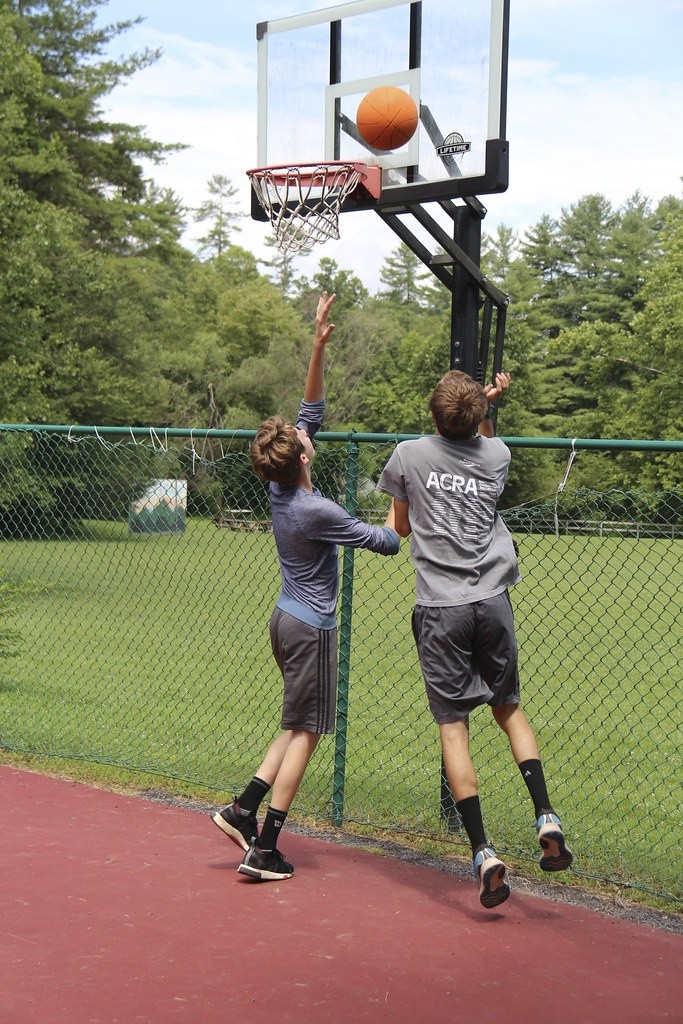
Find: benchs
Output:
[217,525,258,531]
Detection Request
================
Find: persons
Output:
[378,369,574,908]
[210,289,403,881]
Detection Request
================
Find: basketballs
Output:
[357,87,417,149]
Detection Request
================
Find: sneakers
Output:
[472,845,511,910]
[211,793,260,854]
[236,837,296,880]
[534,809,573,873]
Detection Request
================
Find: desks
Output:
[224,518,267,526]
